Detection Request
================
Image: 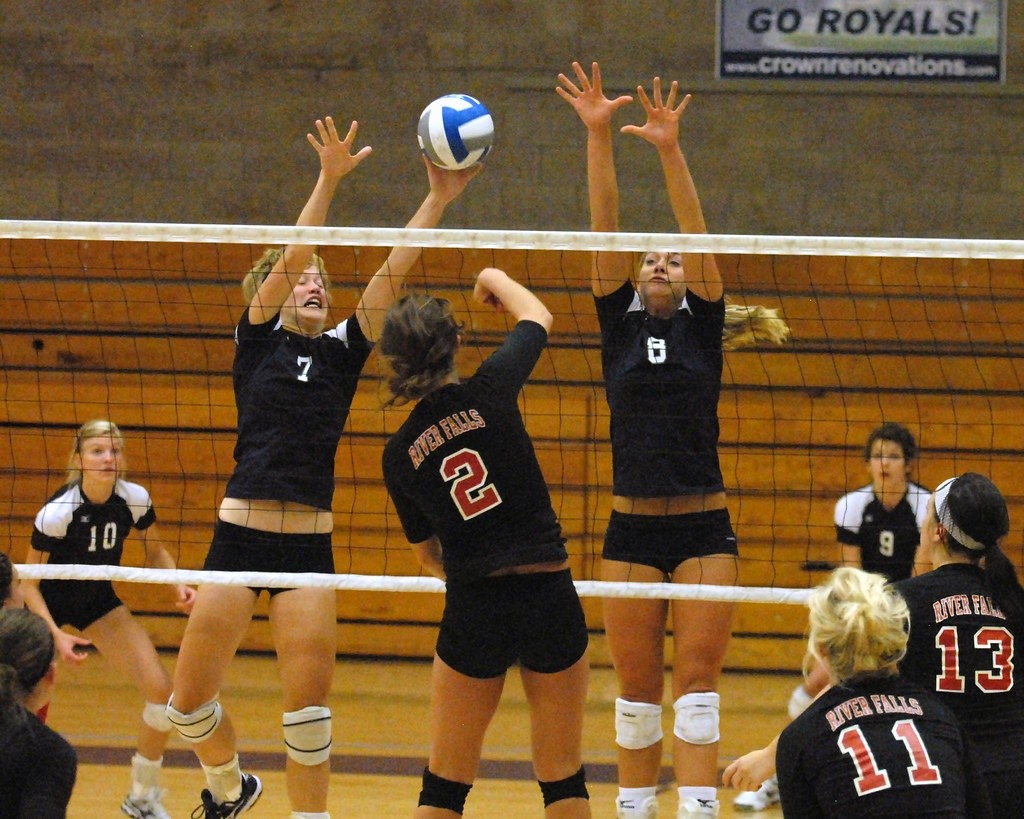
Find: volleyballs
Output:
[417,92,495,170]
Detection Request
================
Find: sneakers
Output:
[120,792,172,819]
[734,778,781,811]
[191,771,262,819]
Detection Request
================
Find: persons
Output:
[721,420,1024,818]
[552,60,742,818]
[0,420,198,819]
[379,267,593,818]
[164,116,484,819]
